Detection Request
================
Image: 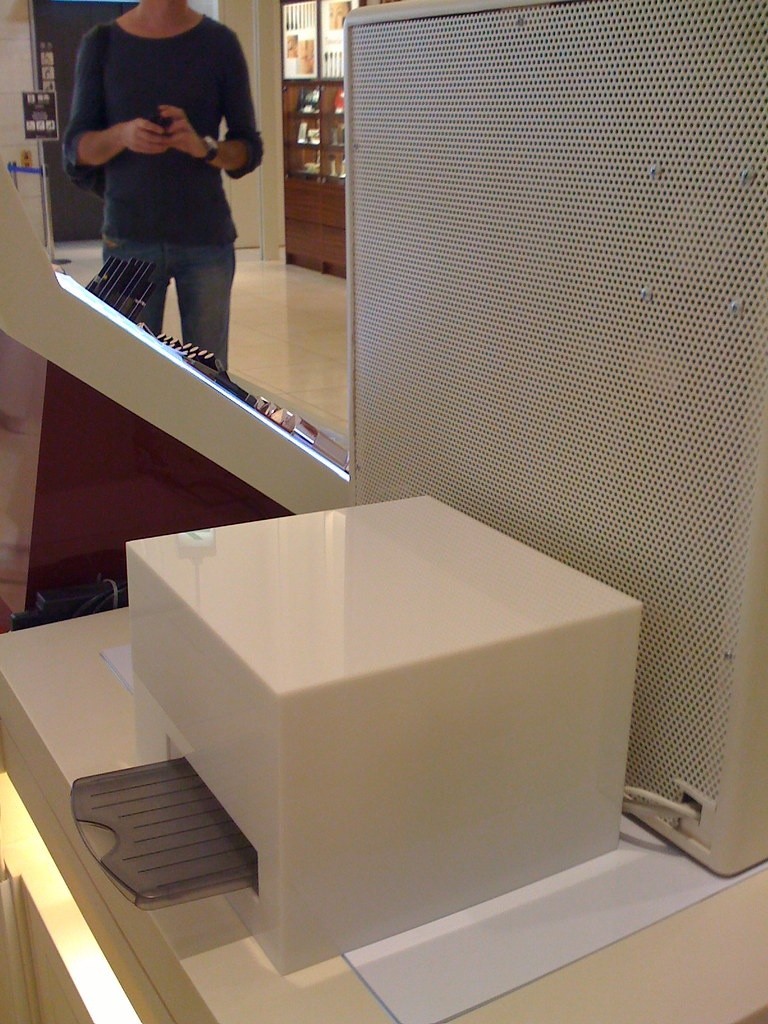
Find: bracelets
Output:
[196,136,218,162]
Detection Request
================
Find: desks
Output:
[1,602,767,1024]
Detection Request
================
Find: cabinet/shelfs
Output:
[279,1,344,281]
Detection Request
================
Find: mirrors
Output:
[0,0,356,477]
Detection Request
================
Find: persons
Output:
[62,0,263,371]
